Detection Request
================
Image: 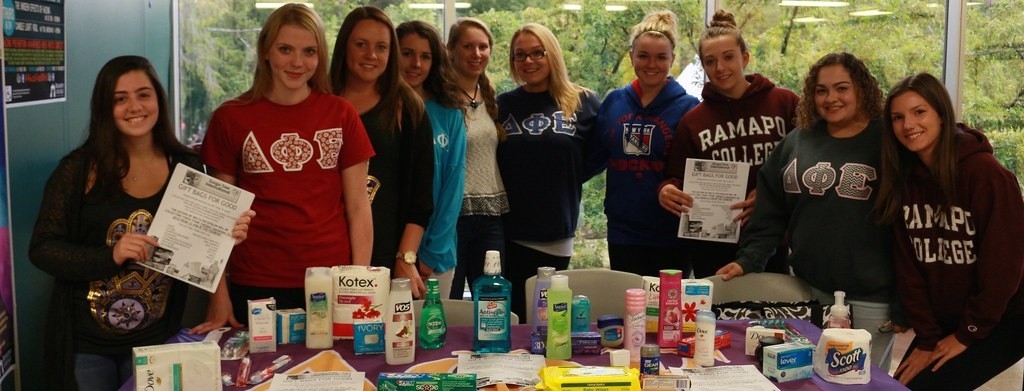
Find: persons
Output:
[28,57,256,390]
[492,22,605,325]
[187,3,376,334]
[326,7,435,300]
[586,10,700,278]
[392,20,468,298]
[715,51,912,379]
[870,71,1024,391]
[657,8,804,283]
[444,16,510,307]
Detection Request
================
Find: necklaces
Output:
[457,82,480,109]
[119,146,159,181]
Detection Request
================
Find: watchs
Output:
[395,250,417,265]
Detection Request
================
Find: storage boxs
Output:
[531,331,602,356]
[745,327,785,356]
[132,340,223,391]
[763,341,814,383]
[676,329,732,359]
[378,372,477,391]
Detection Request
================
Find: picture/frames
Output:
[0,0,67,108]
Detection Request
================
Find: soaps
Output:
[743,326,789,358]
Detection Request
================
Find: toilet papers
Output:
[811,327,876,387]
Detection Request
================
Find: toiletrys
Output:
[304,258,735,391]
[826,287,853,337]
[246,297,280,352]
[130,342,223,390]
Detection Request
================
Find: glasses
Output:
[511,50,548,62]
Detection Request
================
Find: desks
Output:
[118,319,911,391]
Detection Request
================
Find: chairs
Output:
[525,269,646,324]
[703,271,814,322]
[412,299,519,327]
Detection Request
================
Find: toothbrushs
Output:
[222,327,248,361]
[222,374,234,386]
[247,353,294,387]
[235,354,252,389]
